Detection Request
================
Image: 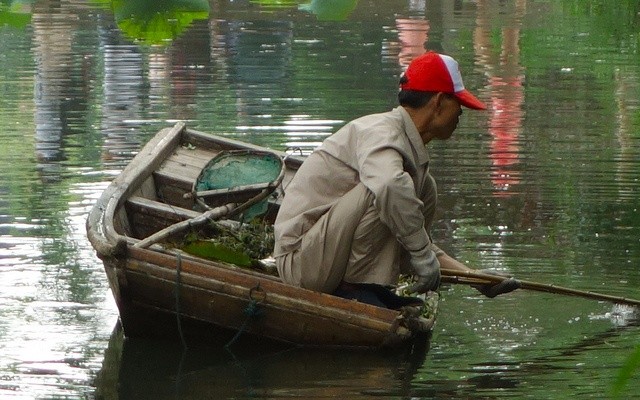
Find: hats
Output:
[399,52,488,110]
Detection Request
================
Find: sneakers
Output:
[377,288,424,309]
[334,287,387,308]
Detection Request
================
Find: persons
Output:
[273,50,518,308]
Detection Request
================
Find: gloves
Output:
[409,240,441,293]
[470,269,520,298]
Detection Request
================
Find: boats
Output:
[85,120,439,354]
